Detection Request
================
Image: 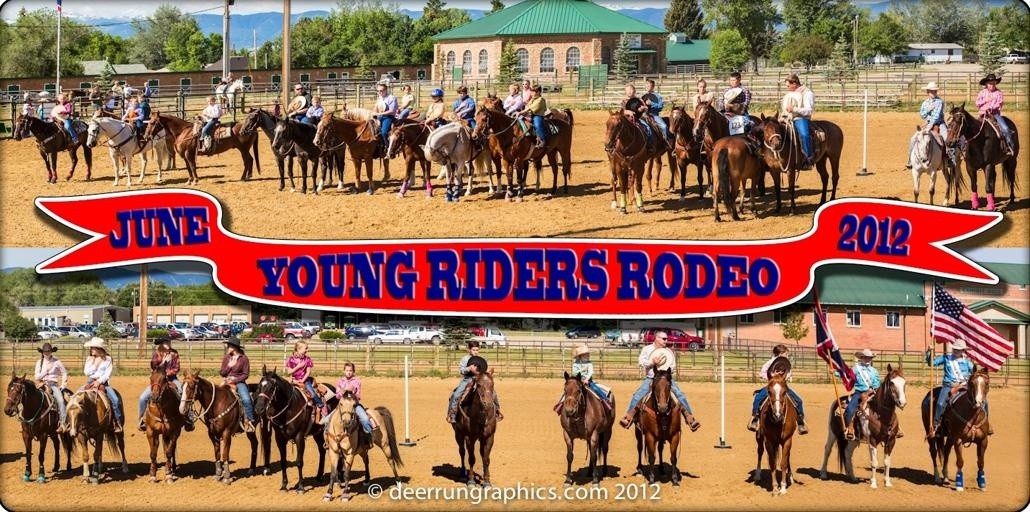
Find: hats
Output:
[980,74,1001,85]
[649,348,674,370]
[948,339,972,350]
[724,88,742,107]
[921,81,939,91]
[222,336,244,348]
[467,356,487,376]
[572,346,593,359]
[290,95,306,112]
[626,98,644,119]
[37,343,57,352]
[84,337,108,351]
[768,356,791,381]
[855,349,876,359]
[782,92,802,116]
[642,94,658,110]
[154,334,175,344]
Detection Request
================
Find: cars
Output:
[616,329,645,349]
[605,328,621,341]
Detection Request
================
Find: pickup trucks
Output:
[1003,53,1028,64]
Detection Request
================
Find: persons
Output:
[976,75,1015,156]
[78,338,122,433]
[22,70,237,156]
[290,77,547,153]
[220,338,255,433]
[138,333,196,431]
[847,350,904,439]
[448,341,504,423]
[35,343,68,432]
[286,341,324,410]
[906,82,955,168]
[622,72,814,164]
[322,362,375,434]
[750,345,808,435]
[572,346,607,401]
[620,330,699,428]
[927,340,993,437]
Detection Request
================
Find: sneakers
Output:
[114,427,122,433]
[56,427,67,433]
[620,419,633,427]
[692,420,699,432]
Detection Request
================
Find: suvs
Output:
[637,326,707,353]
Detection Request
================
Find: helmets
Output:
[430,89,444,97]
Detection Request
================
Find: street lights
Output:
[849,20,856,53]
[855,15,859,53]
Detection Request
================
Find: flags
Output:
[930,284,1014,373]
[811,284,856,390]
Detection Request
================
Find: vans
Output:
[565,323,601,339]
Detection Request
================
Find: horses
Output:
[909,122,968,205]
[2,364,403,503]
[559,371,612,490]
[921,363,990,493]
[451,366,497,490]
[945,101,1020,211]
[820,364,907,489]
[753,368,798,497]
[13,78,573,203]
[634,364,681,490]
[605,95,844,223]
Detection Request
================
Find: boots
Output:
[364,434,372,448]
